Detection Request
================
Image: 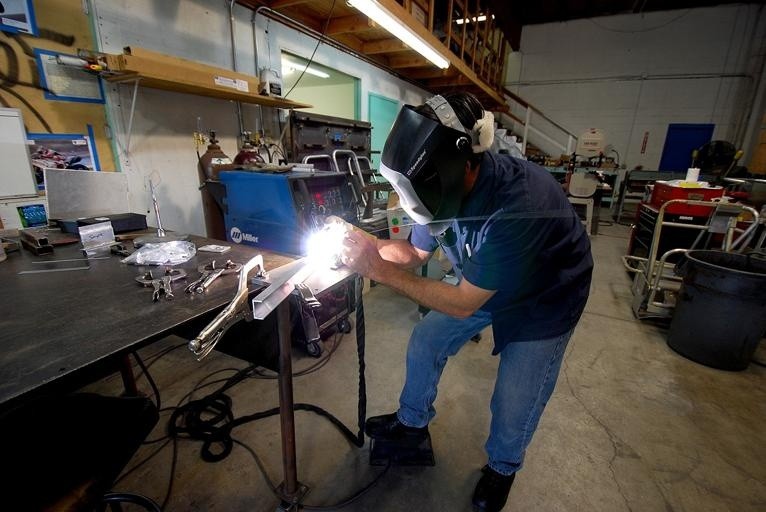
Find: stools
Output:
[567,196,594,236]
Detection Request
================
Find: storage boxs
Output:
[651,180,724,219]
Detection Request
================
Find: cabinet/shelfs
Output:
[614,171,706,226]
[544,166,622,208]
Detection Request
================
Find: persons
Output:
[333,88,595,512]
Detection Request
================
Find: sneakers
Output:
[365,412,429,446]
[471,462,517,511]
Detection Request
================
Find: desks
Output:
[0,227,308,512]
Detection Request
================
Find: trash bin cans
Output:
[665,250,764,372]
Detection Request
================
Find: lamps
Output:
[346,0,450,69]
[452,11,496,25]
[280,60,330,79]
